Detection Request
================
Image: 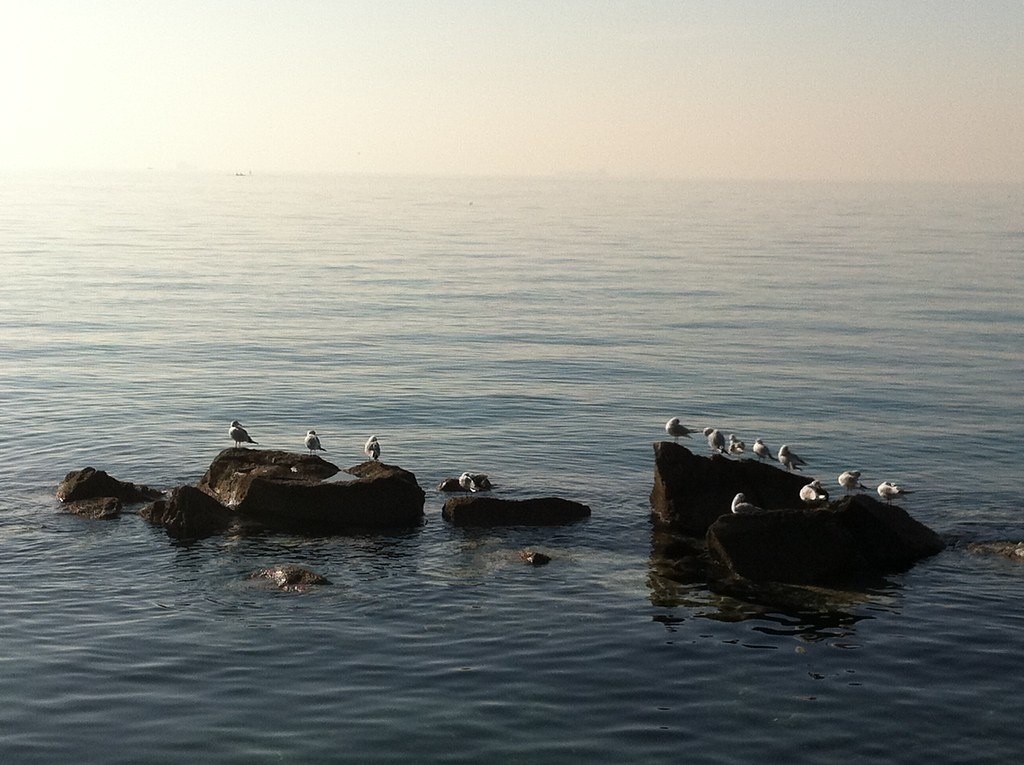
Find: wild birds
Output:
[304,430,327,453]
[229,421,258,448]
[364,435,380,461]
[777,445,806,471]
[459,472,488,496]
[729,434,745,454]
[665,418,699,439]
[730,492,764,514]
[799,480,826,501]
[703,427,729,455]
[752,438,771,461]
[877,482,907,505]
[838,471,871,492]
